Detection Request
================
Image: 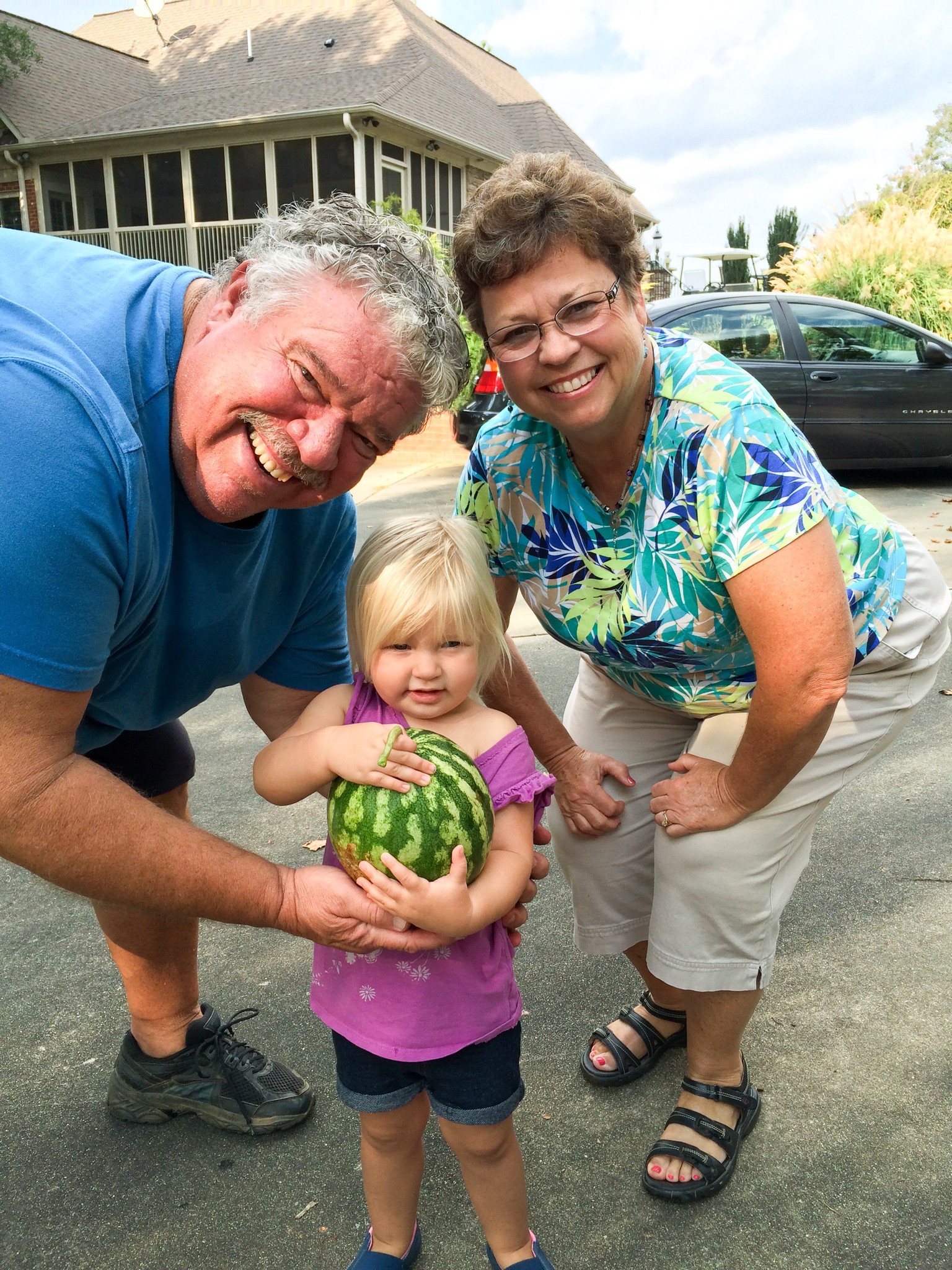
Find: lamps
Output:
[426,140,440,151]
[362,116,379,128]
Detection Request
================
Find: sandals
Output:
[642,1050,761,1205]
[580,988,687,1088]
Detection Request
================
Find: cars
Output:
[450,290,952,472]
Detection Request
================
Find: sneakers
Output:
[107,1003,315,1136]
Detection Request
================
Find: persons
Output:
[451,152,951,1199]
[0,196,552,1134]
[255,516,557,1270]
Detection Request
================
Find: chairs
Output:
[719,328,744,358]
[746,325,775,359]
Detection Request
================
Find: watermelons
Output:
[326,727,494,887]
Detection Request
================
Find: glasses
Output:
[484,274,622,363]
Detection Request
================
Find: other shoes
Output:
[486,1228,556,1270]
[346,1217,422,1270]
